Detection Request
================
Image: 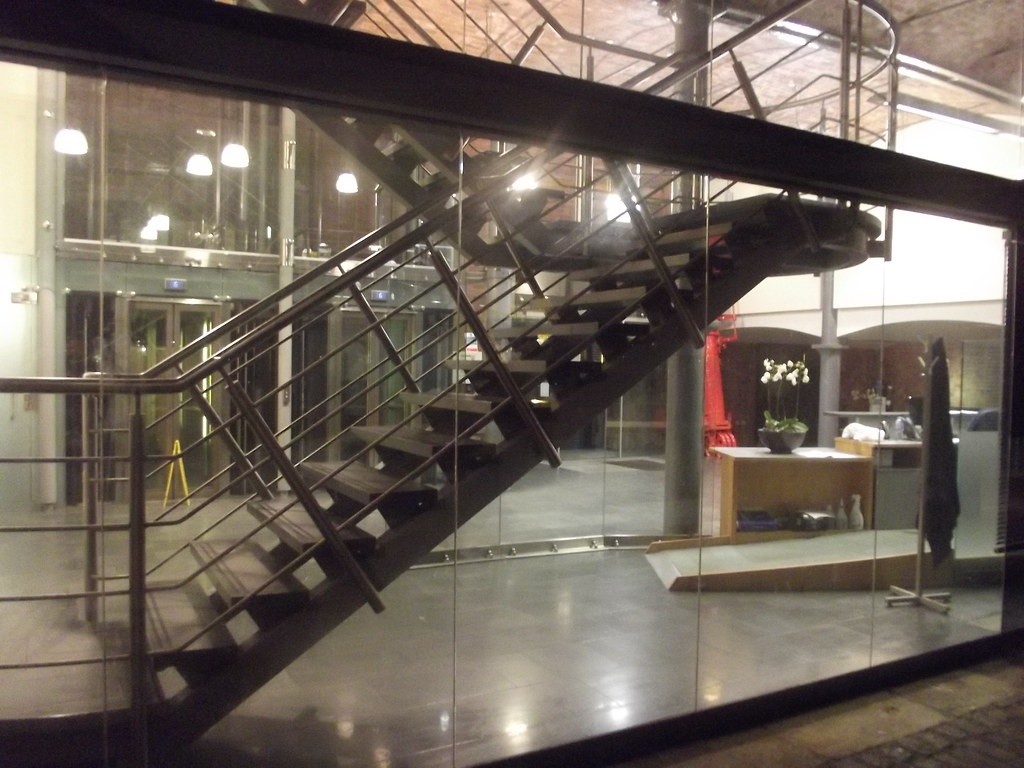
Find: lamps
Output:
[185,92,212,176]
[220,101,249,169]
[336,156,359,194]
[53,75,89,156]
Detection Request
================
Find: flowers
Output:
[759,358,809,433]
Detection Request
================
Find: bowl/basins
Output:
[758,428,806,454]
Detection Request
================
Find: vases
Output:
[757,428,806,455]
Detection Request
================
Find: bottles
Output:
[849,496,864,529]
[824,505,835,529]
[836,504,848,530]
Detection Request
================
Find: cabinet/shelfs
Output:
[708,446,872,538]
[834,438,959,530]
